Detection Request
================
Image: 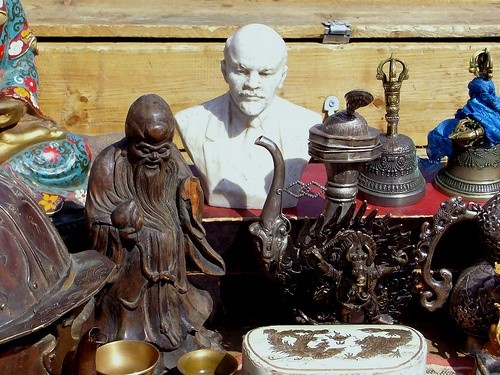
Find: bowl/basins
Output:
[176,349,239,375]
[95,339,160,375]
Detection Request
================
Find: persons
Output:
[174,23,324,209]
[71,94,226,372]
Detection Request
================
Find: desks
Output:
[224,328,456,375]
[190,157,487,223]
